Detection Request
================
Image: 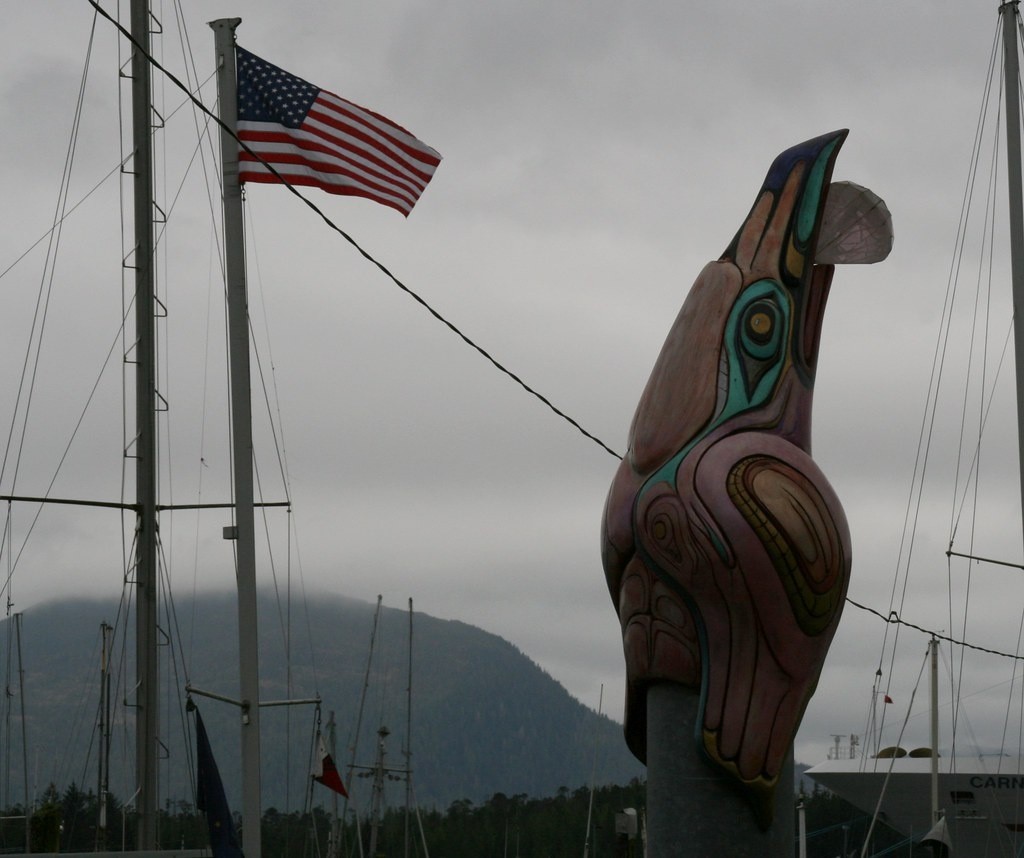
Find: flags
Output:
[195,705,246,858]
[233,42,444,221]
